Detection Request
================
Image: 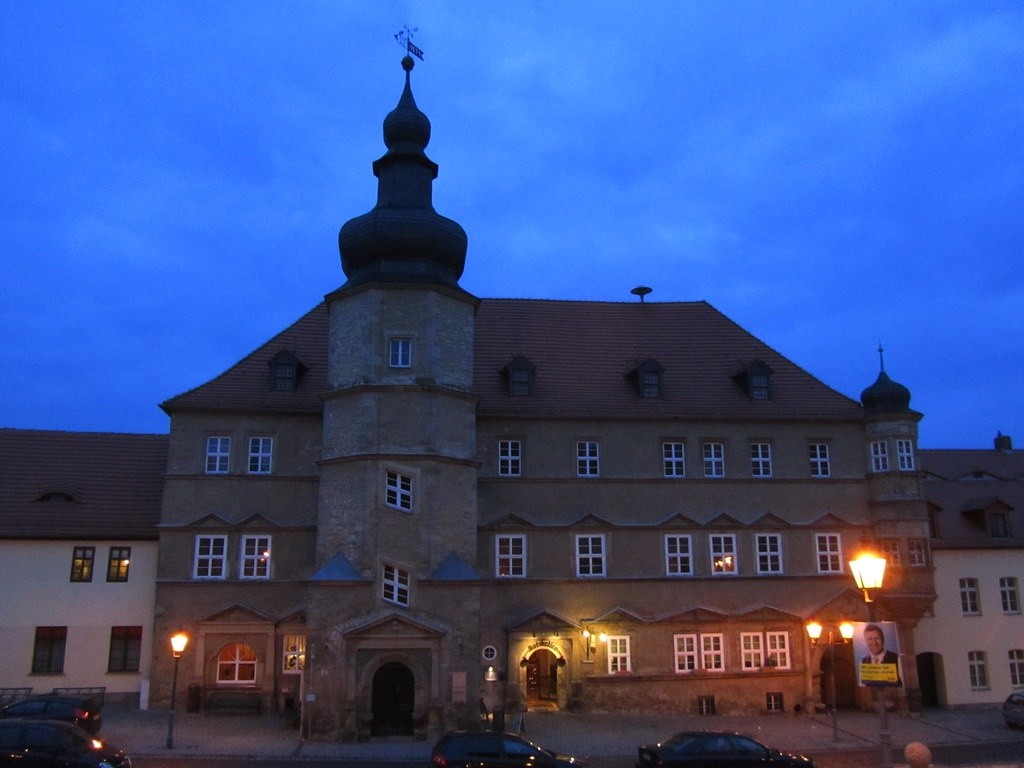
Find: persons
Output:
[858,624,903,687]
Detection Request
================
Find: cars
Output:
[431,729,593,768]
[635,729,818,768]
[1002,691,1024,729]
[0,693,102,735]
[0,719,132,768]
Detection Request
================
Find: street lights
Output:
[849,550,895,768]
[167,633,188,749]
[806,621,856,744]
